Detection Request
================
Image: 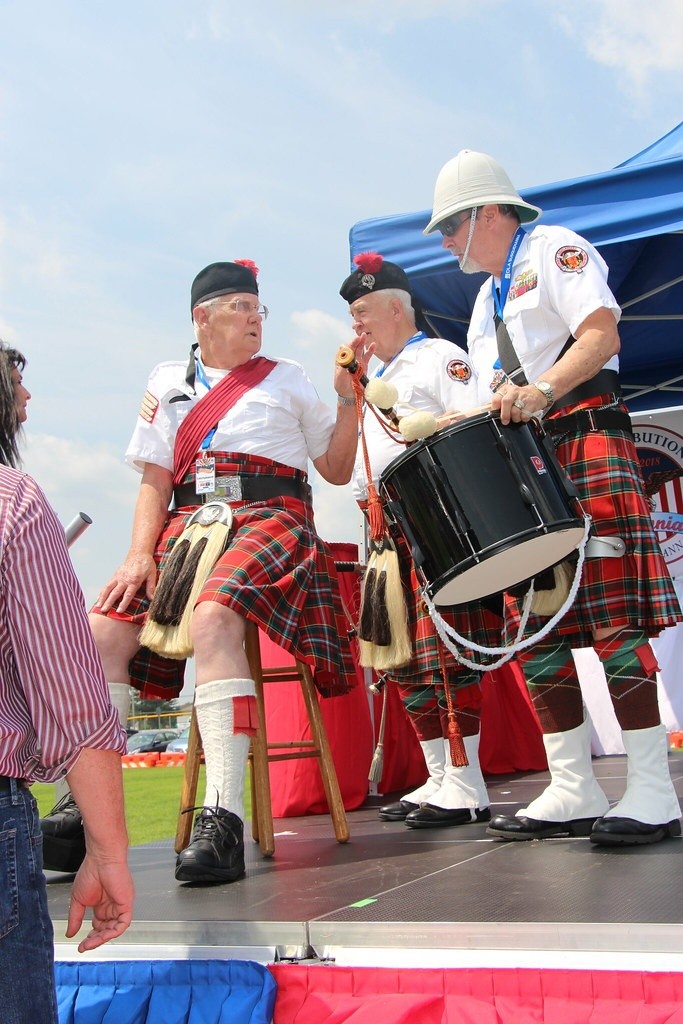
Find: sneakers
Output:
[175,791,246,881]
[38,791,85,870]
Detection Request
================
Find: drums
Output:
[376,410,588,607]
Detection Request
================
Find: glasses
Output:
[441,214,471,237]
[200,300,270,322]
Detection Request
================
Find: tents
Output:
[350,119,683,798]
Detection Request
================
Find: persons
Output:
[340,148,683,847]
[37,263,377,880]
[0,341,135,1024]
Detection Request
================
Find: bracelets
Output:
[338,395,364,406]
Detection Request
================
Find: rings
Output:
[496,391,505,397]
[521,410,533,417]
[514,399,524,409]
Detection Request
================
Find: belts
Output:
[0,776,29,790]
[173,475,312,508]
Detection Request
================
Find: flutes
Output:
[335,347,418,449]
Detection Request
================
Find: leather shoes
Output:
[377,801,420,819]
[486,814,602,840]
[590,817,681,846]
[403,807,491,827]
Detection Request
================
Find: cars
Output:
[126,729,181,755]
[124,729,139,737]
[164,726,192,753]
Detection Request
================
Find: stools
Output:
[175,616,351,860]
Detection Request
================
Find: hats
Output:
[340,254,411,303]
[422,151,543,235]
[190,260,259,323]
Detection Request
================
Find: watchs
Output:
[532,380,554,408]
